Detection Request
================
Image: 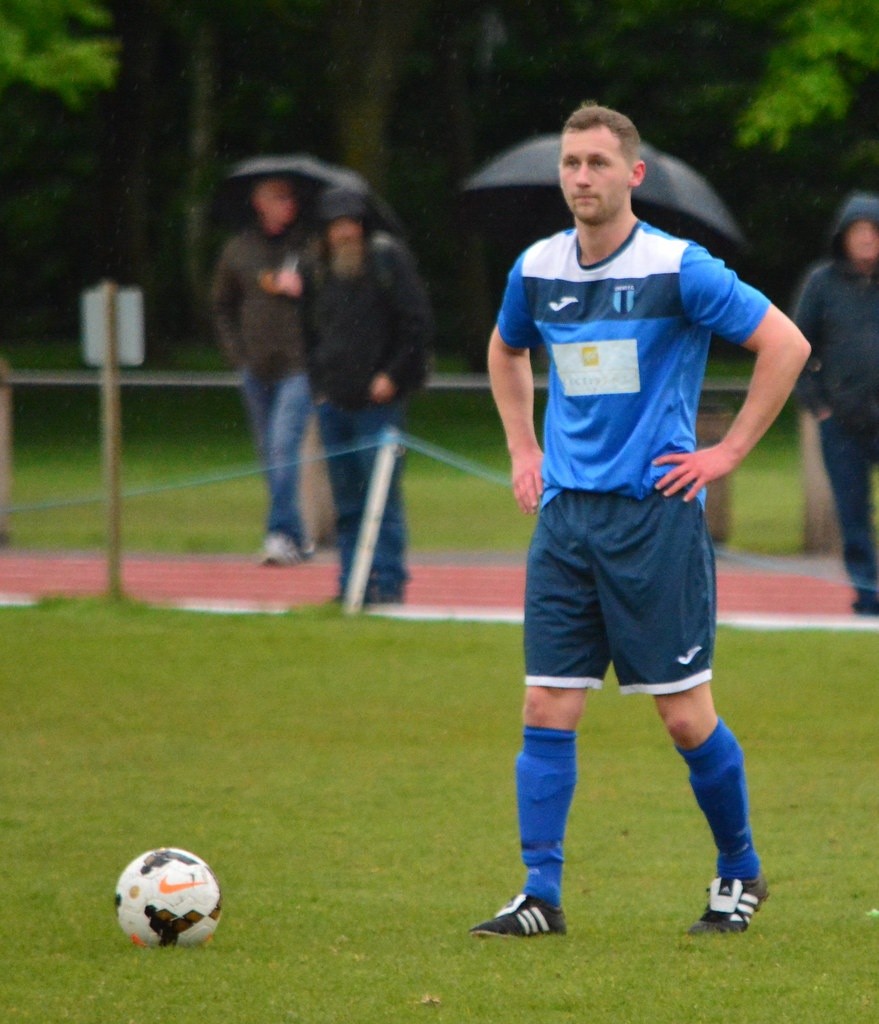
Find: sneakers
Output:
[466,892,565,939]
[687,870,769,934]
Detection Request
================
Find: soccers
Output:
[115,846,224,949]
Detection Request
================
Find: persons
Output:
[468,106,814,941]
[213,176,320,566]
[270,187,428,609]
[791,195,878,618]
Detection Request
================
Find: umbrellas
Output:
[219,158,398,291]
[466,135,751,282]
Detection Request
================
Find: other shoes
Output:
[261,534,297,566]
